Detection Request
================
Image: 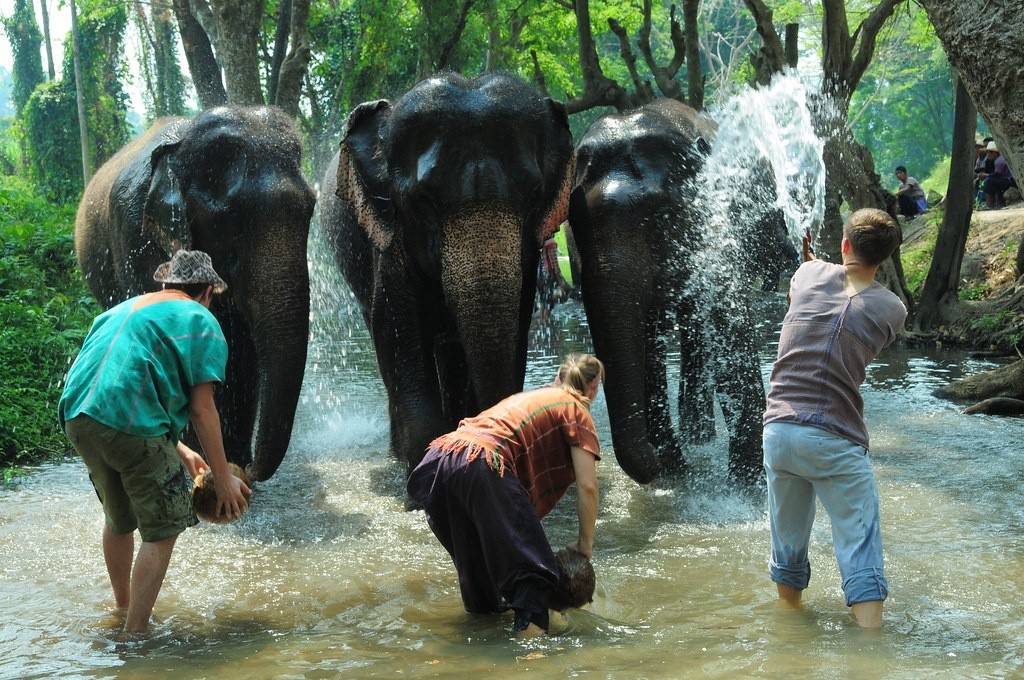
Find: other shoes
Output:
[994,202,1006,210]
[983,205,993,210]
[905,213,913,221]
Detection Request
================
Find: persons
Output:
[59,250,251,630]
[404,353,605,635]
[892,166,927,221]
[763,207,908,627]
[973,138,1016,210]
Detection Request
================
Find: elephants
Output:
[74,103,318,482]
[568,95,802,509]
[322,68,576,516]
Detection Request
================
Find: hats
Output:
[152,250,227,294]
[975,141,983,146]
[978,141,999,152]
